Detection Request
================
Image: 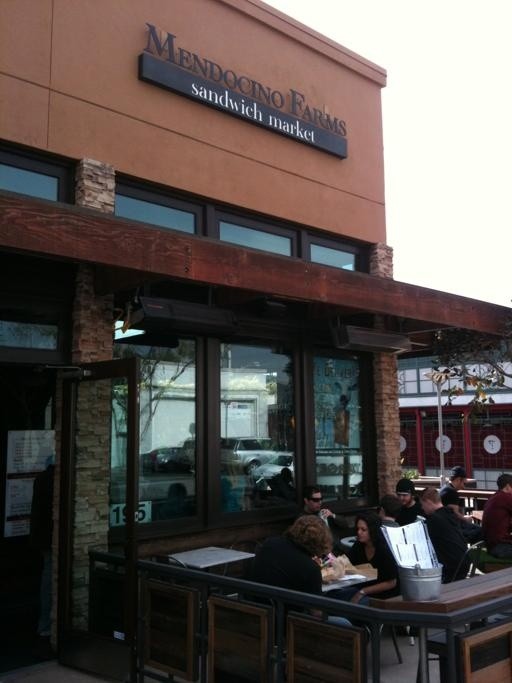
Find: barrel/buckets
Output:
[399,562,444,602]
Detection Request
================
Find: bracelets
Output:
[359,589,366,595]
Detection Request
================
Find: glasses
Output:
[310,497,323,502]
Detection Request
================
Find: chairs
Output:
[451,540,487,581]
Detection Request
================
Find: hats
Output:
[396,479,415,495]
[448,466,467,477]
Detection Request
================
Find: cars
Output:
[107,431,295,504]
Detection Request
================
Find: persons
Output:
[480,472,511,561]
[152,466,297,521]
[333,511,402,627]
[438,465,486,544]
[289,485,350,561]
[417,486,471,584]
[238,515,354,648]
[391,477,426,526]
[371,492,402,527]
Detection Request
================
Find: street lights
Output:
[425,370,454,488]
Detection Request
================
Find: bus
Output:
[275,353,364,502]
